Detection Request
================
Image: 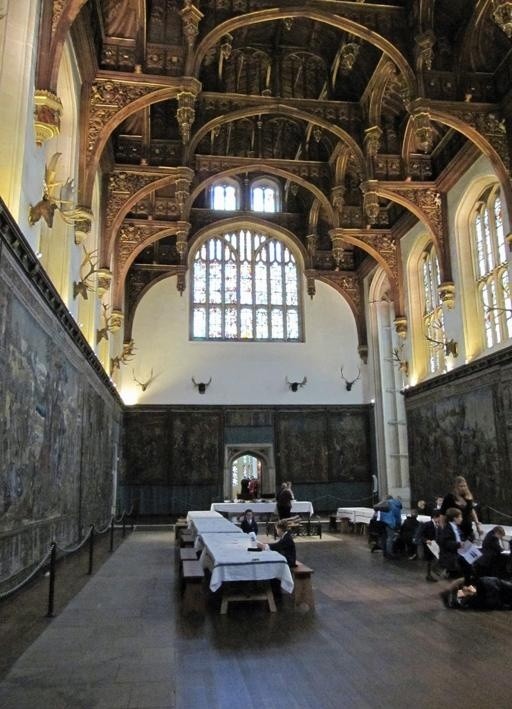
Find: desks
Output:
[186,510,295,615]
[211,501,314,537]
[337,506,431,536]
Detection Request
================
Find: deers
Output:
[285,376,306,392]
[29,153,87,228]
[192,378,211,394]
[97,302,121,343]
[424,334,456,356]
[384,348,408,373]
[341,368,360,391]
[132,369,153,391]
[110,351,136,378]
[73,245,112,300]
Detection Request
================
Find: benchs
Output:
[173,518,205,621]
[256,535,315,615]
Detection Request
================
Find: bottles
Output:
[248,531,257,547]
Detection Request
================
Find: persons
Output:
[241,509,258,536]
[276,481,294,519]
[257,520,297,568]
[373,476,512,608]
[241,475,257,499]
